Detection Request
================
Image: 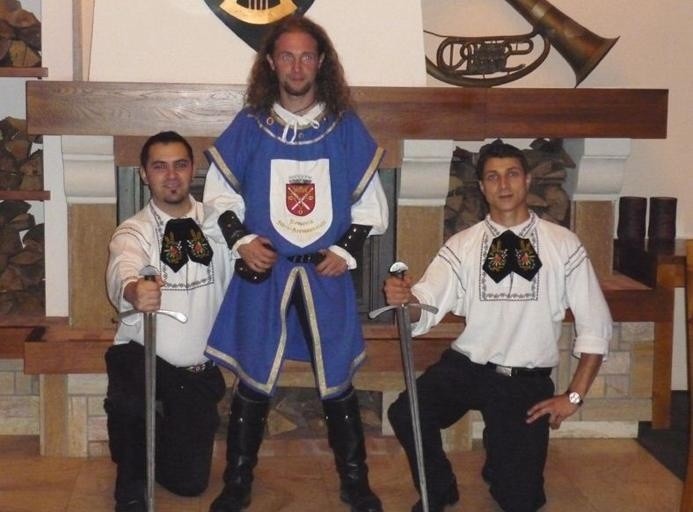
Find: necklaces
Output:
[291,94,316,117]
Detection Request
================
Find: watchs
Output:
[564,385,586,410]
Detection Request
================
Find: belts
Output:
[448,343,554,379]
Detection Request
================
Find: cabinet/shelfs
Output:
[0,66,68,359]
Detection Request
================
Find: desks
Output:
[613,238,693,432]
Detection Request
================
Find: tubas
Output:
[422,1,621,90]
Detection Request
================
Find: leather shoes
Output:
[114,493,146,512]
[409,479,462,512]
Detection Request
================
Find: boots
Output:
[206,383,274,512]
[314,384,383,512]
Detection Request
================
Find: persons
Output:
[104,129,236,511]
[382,136,616,512]
[195,14,389,510]
